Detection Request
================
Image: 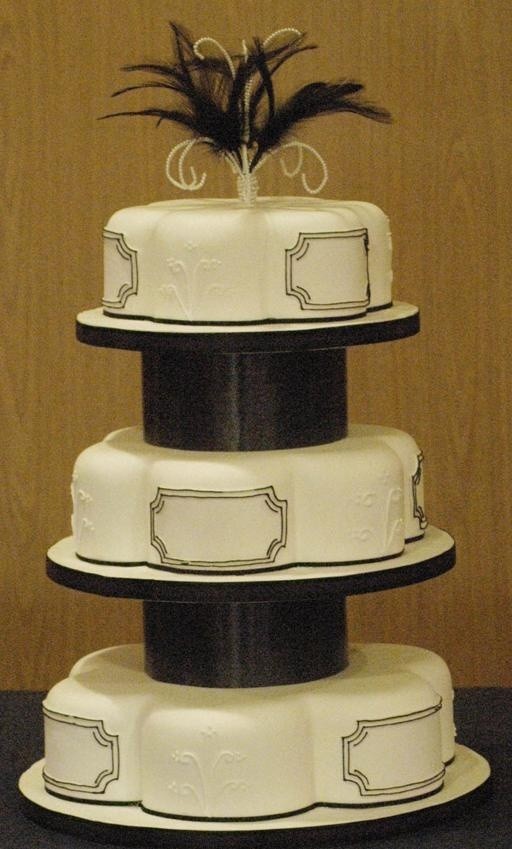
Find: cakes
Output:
[41,640,456,824]
[70,420,427,574]
[103,195,394,325]
